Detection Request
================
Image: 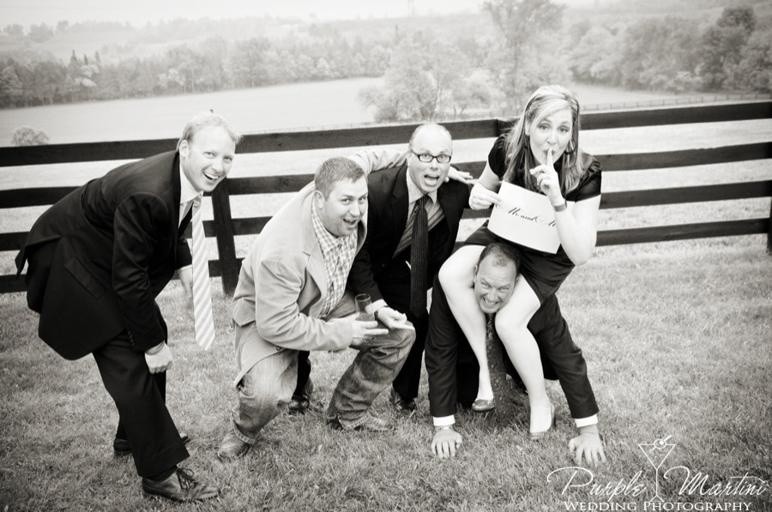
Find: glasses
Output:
[407,149,456,165]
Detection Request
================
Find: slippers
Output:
[285,381,314,411]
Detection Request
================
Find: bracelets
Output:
[553,200,568,213]
[433,426,453,433]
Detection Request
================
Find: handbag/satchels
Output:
[486,182,566,258]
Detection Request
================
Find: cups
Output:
[355,294,375,323]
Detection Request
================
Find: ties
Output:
[188,196,220,349]
[408,198,432,318]
[484,314,515,427]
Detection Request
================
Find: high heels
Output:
[471,392,498,413]
[526,400,556,439]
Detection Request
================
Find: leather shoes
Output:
[388,387,419,419]
[327,412,394,435]
[140,472,220,504]
[503,374,526,407]
[217,420,254,460]
[110,427,189,461]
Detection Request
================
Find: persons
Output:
[213,145,478,460]
[438,84,603,441]
[423,240,609,467]
[342,120,475,415]
[13,108,247,503]
